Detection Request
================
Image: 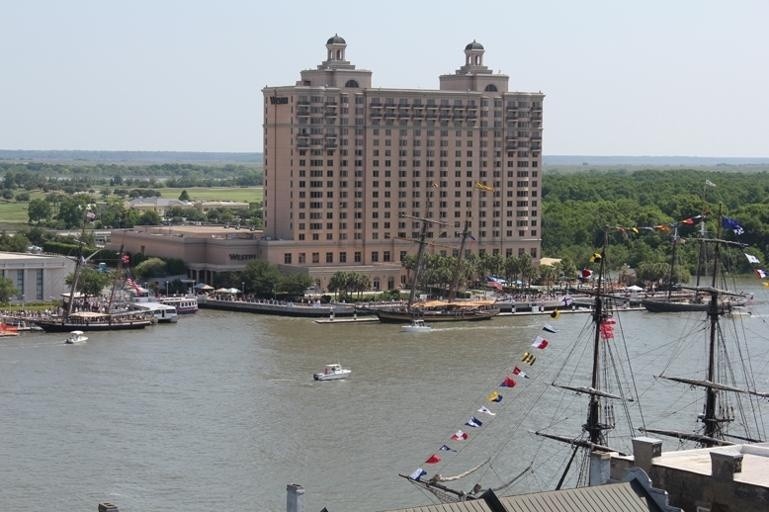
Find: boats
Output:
[310,358,354,383]
[398,316,433,334]
[0,207,200,345]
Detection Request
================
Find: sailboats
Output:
[637,177,755,314]
[351,179,502,325]
[396,201,768,506]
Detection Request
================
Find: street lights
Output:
[241,282,245,293]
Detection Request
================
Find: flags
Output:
[533,253,601,351]
[430,183,439,193]
[412,416,483,479]
[478,352,537,416]
[615,209,768,287]
[486,276,502,290]
[473,182,494,193]
